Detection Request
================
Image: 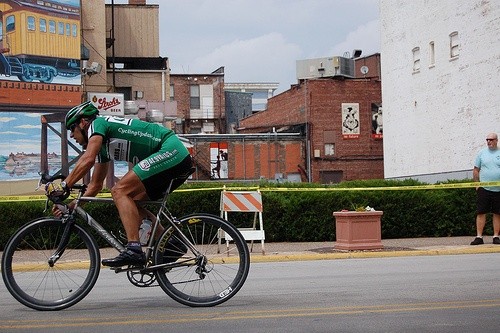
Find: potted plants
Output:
[333,199,384,249]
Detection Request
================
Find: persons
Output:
[45,101,193,269]
[210,155,221,179]
[470,133,500,245]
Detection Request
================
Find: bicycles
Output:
[0,171,251,311]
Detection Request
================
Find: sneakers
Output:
[162,241,187,264]
[102,244,147,267]
[470,237,484,245]
[492,237,500,244]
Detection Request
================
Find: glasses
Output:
[486,139,496,141]
[69,119,89,132]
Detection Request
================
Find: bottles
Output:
[139,216,153,245]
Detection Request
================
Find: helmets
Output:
[65,101,99,130]
